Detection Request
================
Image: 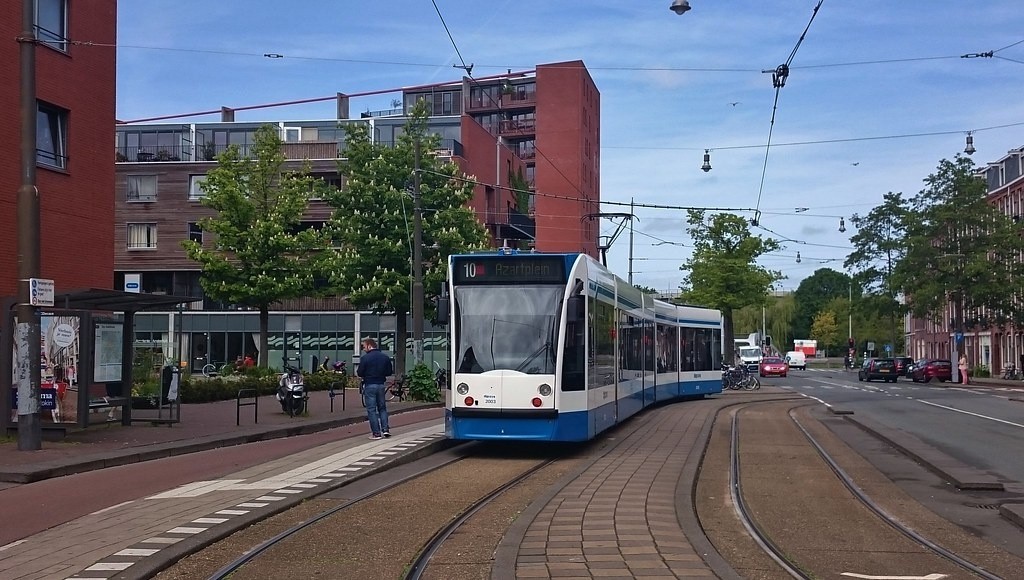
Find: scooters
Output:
[275,365,304,416]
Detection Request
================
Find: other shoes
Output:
[384,432,392,437]
[368,436,382,440]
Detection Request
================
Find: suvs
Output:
[858,356,950,384]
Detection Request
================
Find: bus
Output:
[436,212,722,443]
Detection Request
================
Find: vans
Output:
[785,351,807,371]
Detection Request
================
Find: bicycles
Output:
[202,360,233,378]
[385,369,416,402]
[720,361,761,391]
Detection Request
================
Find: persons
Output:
[107,407,117,421]
[1002,362,1015,380]
[235,356,243,373]
[67,366,76,387]
[357,339,393,440]
[238,354,254,369]
[844,355,851,370]
[958,354,968,384]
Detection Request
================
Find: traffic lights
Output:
[765,335,771,346]
[849,339,854,348]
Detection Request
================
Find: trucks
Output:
[734,338,763,372]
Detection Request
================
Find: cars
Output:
[760,357,787,378]
